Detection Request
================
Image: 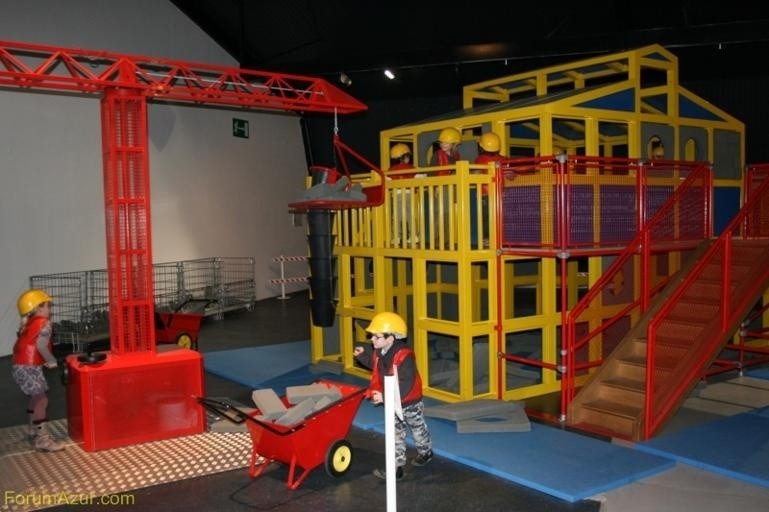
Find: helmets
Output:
[18,290,53,315]
[553,146,564,155]
[390,143,411,159]
[438,128,461,144]
[365,312,408,339]
[652,146,664,156]
[479,133,500,153]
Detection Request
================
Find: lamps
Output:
[339,65,405,86]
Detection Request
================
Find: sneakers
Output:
[30,428,56,441]
[411,448,433,466]
[34,432,65,451]
[373,466,403,481]
